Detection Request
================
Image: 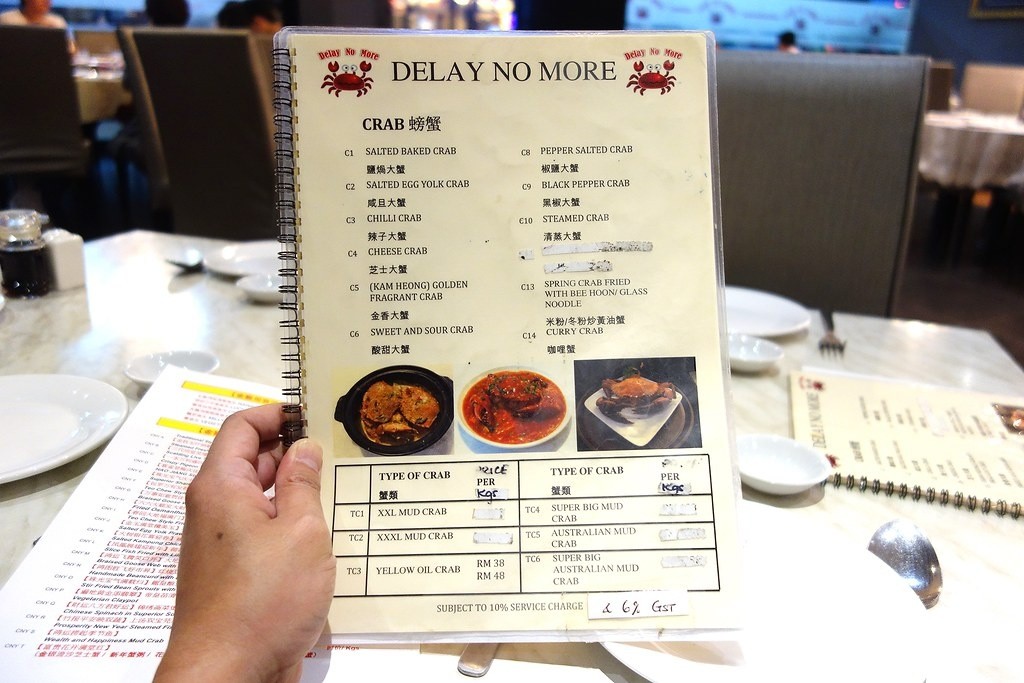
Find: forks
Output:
[818,308,844,361]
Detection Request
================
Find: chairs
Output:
[72,28,119,53]
[715,48,931,315]
[116,24,285,241]
[0,22,89,213]
[961,63,1024,113]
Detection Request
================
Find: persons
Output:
[144,0,189,29]
[779,32,800,53]
[150,402,337,683]
[0,0,75,54]
[217,0,283,33]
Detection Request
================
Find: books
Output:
[272,26,754,642]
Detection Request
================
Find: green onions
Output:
[481,375,546,429]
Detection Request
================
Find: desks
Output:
[917,107,1024,262]
[74,66,132,141]
[0,229,1024,683]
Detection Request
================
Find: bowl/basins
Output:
[456,365,572,449]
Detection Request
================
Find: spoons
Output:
[167,247,202,271]
[867,519,942,606]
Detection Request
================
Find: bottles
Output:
[0,209,50,298]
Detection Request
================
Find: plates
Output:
[236,274,284,303]
[122,349,220,389]
[728,335,784,372]
[203,240,287,277]
[598,518,929,683]
[736,434,831,496]
[584,376,682,446]
[0,374,128,485]
[726,286,811,337]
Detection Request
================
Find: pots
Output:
[334,365,454,456]
